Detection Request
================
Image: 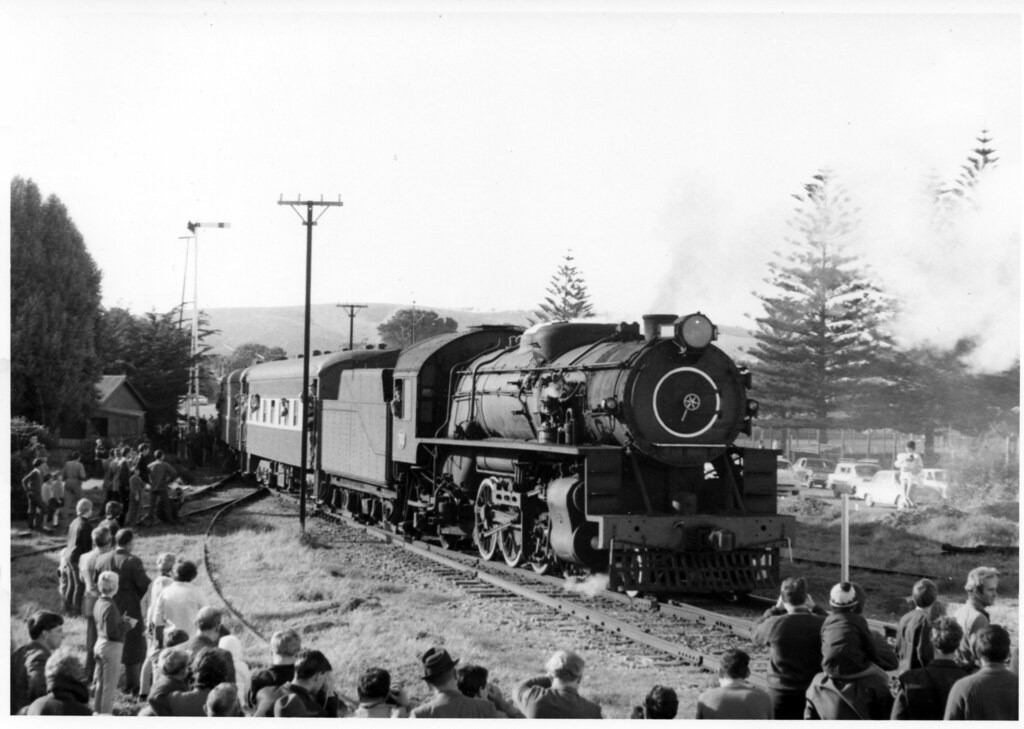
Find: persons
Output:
[390,388,402,418]
[233,392,260,419]
[281,397,288,416]
[630,684,680,720]
[893,440,924,509]
[511,649,605,719]
[10,417,514,719]
[750,563,1019,721]
[308,384,316,396]
[694,648,774,720]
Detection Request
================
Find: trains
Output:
[214,309,798,601]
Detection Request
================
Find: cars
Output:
[777,454,800,496]
[921,469,950,497]
[863,470,946,509]
[828,461,883,501]
[792,457,837,489]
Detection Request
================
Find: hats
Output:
[71,450,83,458]
[827,582,864,608]
[906,441,916,450]
[548,648,585,676]
[137,442,150,451]
[418,646,460,680]
[274,693,316,717]
[104,501,124,520]
[33,457,46,466]
